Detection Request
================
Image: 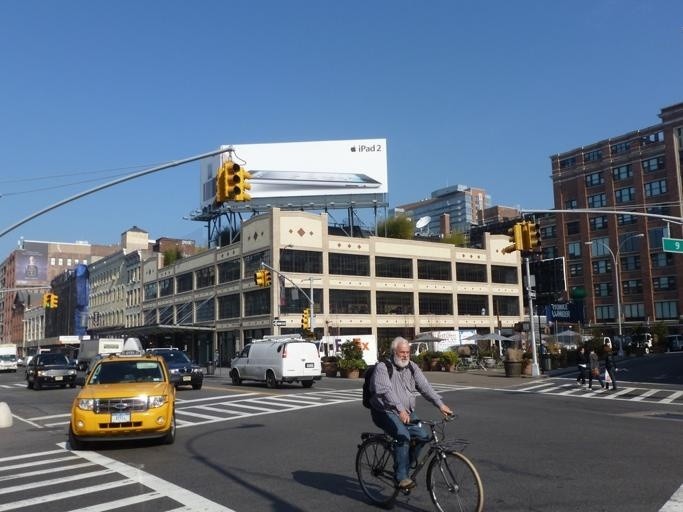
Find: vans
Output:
[227,338,321,388]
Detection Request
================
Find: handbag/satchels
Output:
[591,367,600,377]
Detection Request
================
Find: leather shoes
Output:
[396,479,418,488]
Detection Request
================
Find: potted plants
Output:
[322,341,367,378]
[410,350,458,373]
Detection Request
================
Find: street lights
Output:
[584,234,644,335]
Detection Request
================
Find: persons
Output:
[575,347,588,387]
[603,345,617,390]
[585,348,605,389]
[370,335,455,489]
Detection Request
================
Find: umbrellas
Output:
[462,334,481,341]
[409,335,446,342]
[508,334,519,341]
[476,332,512,356]
[555,330,579,348]
[540,333,548,338]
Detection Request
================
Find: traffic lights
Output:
[255,268,271,286]
[42,293,56,308]
[300,309,309,330]
[213,161,249,205]
[507,221,541,256]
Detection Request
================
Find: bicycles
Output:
[355,414,484,512]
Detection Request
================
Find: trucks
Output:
[406,330,477,361]
[73,338,124,371]
[309,334,377,369]
[0,344,17,372]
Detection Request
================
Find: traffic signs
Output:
[273,321,285,326]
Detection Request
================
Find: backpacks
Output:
[362,359,415,409]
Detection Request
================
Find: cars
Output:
[69,355,176,449]
[143,347,202,392]
[580,333,683,354]
[26,353,77,390]
